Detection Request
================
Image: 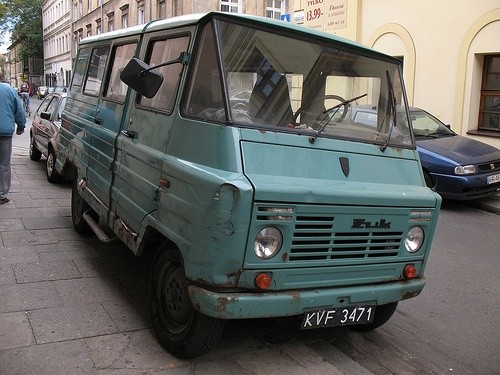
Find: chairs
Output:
[249,73,296,128]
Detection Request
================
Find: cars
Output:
[331,96,500,210]
[28,91,69,183]
[35,85,69,100]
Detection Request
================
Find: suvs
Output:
[52,9,444,361]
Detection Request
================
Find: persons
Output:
[0,67,31,206]
